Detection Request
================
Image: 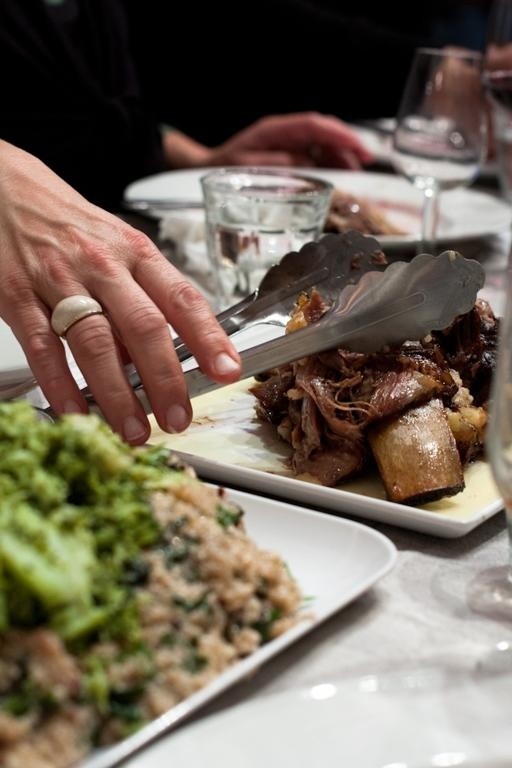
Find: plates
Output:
[14,308,511,538]
[61,482,398,767]
[125,166,415,219]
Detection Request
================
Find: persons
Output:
[0,0,375,448]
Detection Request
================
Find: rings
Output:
[49,293,104,339]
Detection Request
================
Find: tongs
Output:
[37,228,486,431]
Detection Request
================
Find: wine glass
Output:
[394,46,490,255]
[463,251,511,621]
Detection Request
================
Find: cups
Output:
[199,159,336,301]
